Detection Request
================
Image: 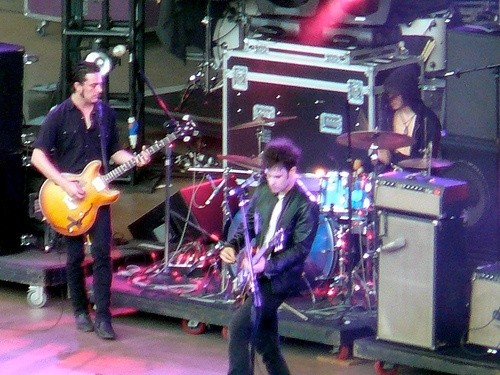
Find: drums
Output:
[225,203,340,295]
[296,173,322,200]
[317,171,371,221]
[439,159,490,230]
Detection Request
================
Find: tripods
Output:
[173,0,233,114]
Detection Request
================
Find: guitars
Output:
[39,114,201,237]
[227,228,284,300]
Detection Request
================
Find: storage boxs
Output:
[221,46,422,177]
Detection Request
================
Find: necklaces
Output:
[395,111,416,136]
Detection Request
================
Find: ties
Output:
[263,195,285,246]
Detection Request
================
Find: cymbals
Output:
[228,115,298,131]
[337,131,416,151]
[217,154,263,172]
[398,158,452,169]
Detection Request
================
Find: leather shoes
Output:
[76,313,94,332]
[94,319,115,339]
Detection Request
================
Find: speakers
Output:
[467,263,499,351]
[437,140,499,261]
[443,27,499,143]
[378,212,470,350]
[0,44,30,257]
[129,191,204,243]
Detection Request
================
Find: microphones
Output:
[382,238,406,253]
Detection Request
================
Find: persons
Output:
[30,59,152,340]
[219,138,323,375]
[352,66,443,174]
